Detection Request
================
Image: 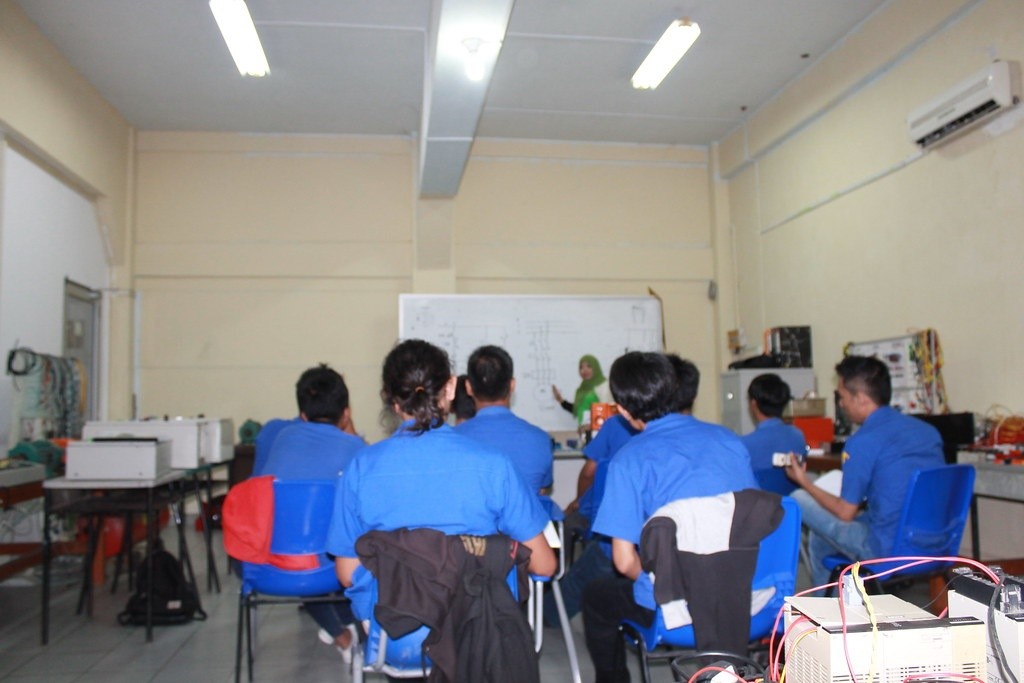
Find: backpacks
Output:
[114,534,208,626]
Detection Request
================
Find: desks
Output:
[39,460,234,648]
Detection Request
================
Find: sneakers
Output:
[317,627,334,645]
[338,624,360,665]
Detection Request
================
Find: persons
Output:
[245,366,367,666]
[523,356,699,629]
[553,355,613,441]
[785,357,950,597]
[328,338,558,681]
[580,351,762,683]
[455,346,553,499]
[740,374,803,496]
[451,376,476,425]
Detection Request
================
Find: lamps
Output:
[208,0,271,79]
[629,17,702,91]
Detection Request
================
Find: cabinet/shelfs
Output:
[970,464,1024,562]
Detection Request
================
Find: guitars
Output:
[771,450,842,473]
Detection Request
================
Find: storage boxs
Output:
[82,419,215,469]
[957,450,986,466]
[763,325,813,368]
[200,419,236,464]
[782,399,826,418]
[783,416,836,448]
[65,439,172,480]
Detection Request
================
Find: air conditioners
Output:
[908,58,1018,150]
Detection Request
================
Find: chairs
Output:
[232,477,368,683]
[353,572,551,683]
[819,462,977,598]
[622,493,804,683]
[549,501,584,683]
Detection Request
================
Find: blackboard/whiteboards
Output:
[397,294,663,430]
